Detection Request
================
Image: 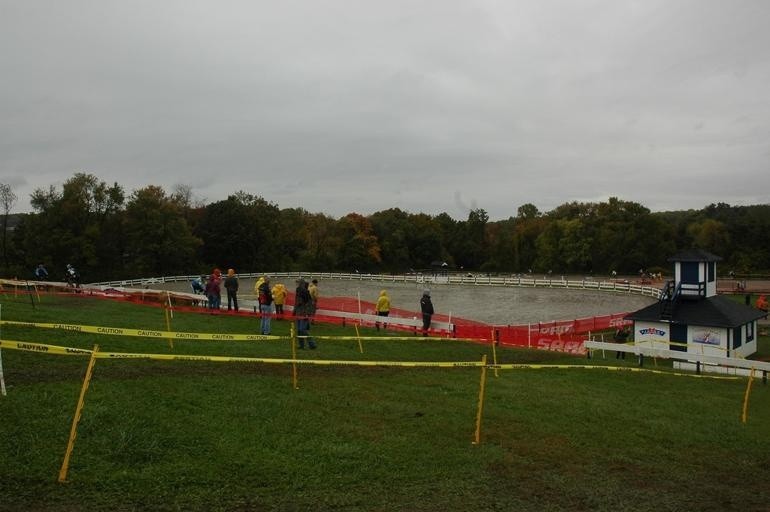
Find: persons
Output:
[255,275,320,350]
[64,263,81,292]
[419,288,435,337]
[191,267,223,310]
[637,267,664,286]
[34,263,49,290]
[374,288,391,332]
[612,323,631,361]
[223,268,240,312]
